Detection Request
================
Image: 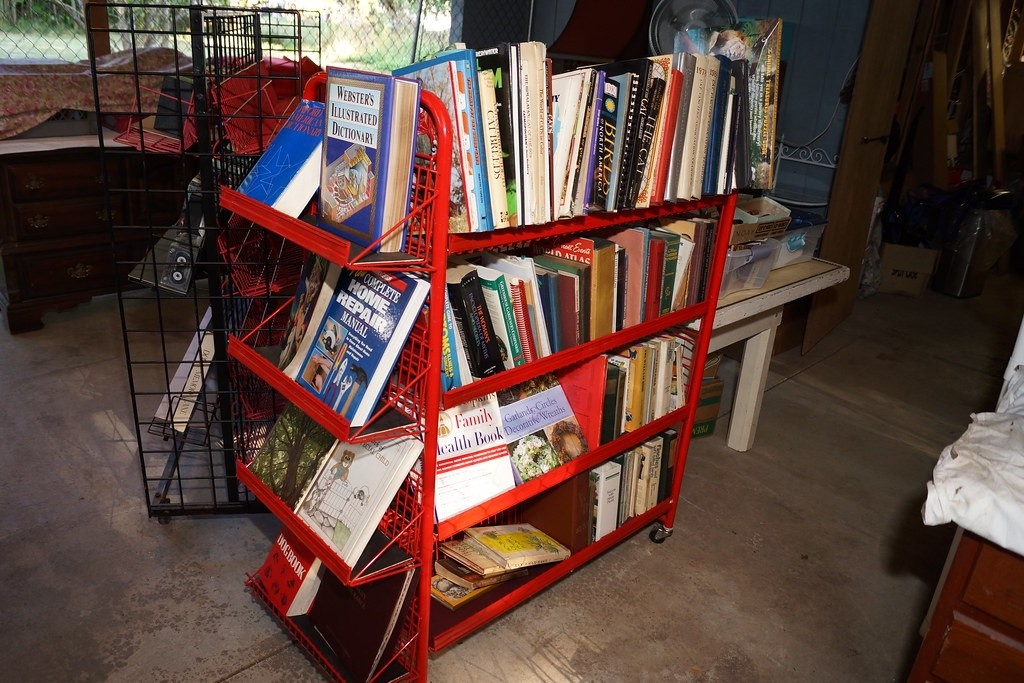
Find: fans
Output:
[647,0,738,61]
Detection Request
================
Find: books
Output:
[391,16,784,235]
[430,522,572,613]
[272,249,432,428]
[257,523,323,618]
[440,214,719,392]
[235,65,423,256]
[245,401,426,573]
[433,331,687,527]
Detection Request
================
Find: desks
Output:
[690,257,854,454]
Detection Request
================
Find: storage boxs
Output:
[734,195,791,224]
[686,350,727,439]
[876,240,944,299]
[730,216,793,246]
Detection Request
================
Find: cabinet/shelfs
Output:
[217,71,740,683]
[0,134,216,335]
[905,521,1024,683]
